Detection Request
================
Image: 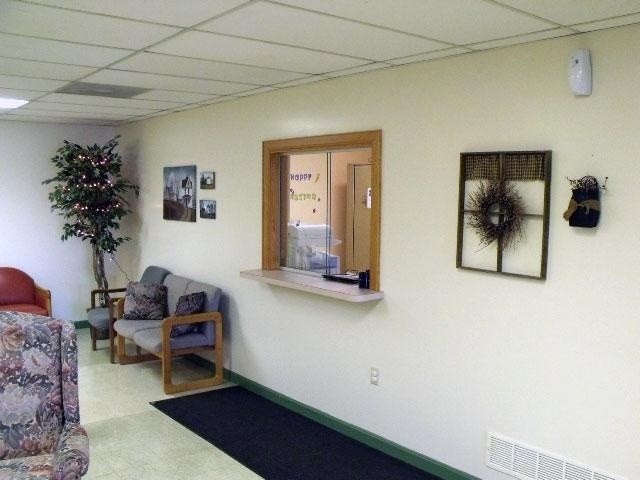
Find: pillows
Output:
[170,292,208,335]
[122,281,166,320]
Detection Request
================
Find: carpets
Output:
[148,383,478,479]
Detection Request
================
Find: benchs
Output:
[114,275,223,396]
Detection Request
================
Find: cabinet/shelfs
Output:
[346,163,370,274]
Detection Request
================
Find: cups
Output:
[358,272,370,289]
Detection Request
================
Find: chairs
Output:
[1,266,50,321]
[87,266,171,363]
[2,310,87,480]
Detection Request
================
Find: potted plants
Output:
[41,133,142,341]
